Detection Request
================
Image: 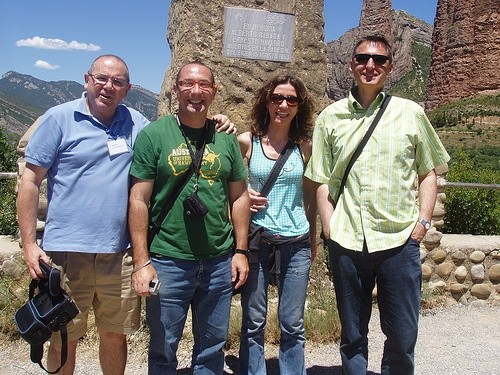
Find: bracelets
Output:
[235,249,247,255]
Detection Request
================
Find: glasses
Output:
[353,54,390,64]
[178,80,213,89]
[270,93,302,107]
[89,73,128,88]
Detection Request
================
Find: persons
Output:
[17,55,237,375]
[304,36,451,375]
[130,61,249,375]
[238,74,318,375]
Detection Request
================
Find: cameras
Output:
[149,279,161,296]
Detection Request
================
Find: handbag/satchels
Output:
[16,275,81,373]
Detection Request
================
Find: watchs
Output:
[418,218,431,230]
